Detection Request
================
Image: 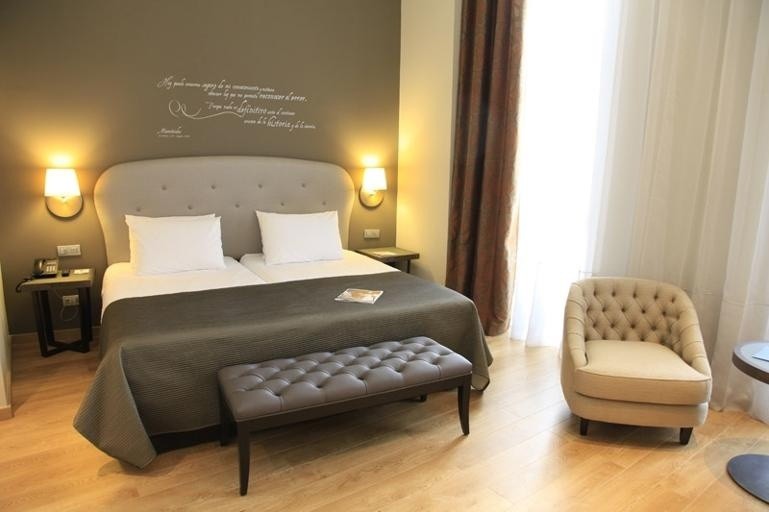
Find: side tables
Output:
[722,339,768,507]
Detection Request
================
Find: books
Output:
[334,288,384,304]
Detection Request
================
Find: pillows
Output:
[253,208,347,267]
[124,216,227,277]
[124,212,217,268]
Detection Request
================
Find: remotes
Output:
[61,267,70,276]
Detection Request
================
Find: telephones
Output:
[34,257,59,277]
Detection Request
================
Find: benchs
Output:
[214,334,475,499]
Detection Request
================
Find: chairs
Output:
[558,275,715,446]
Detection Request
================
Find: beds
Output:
[71,155,493,473]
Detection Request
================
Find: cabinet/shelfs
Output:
[15,267,96,359]
[360,245,420,274]
